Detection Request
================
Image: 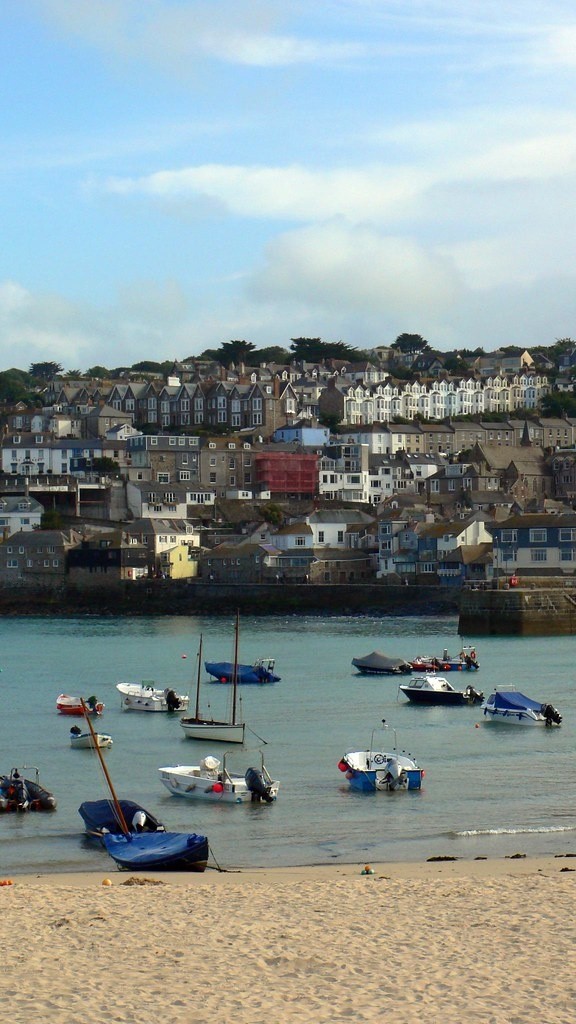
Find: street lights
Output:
[493,535,500,590]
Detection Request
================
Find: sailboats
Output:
[180,607,246,744]
[78,696,209,873]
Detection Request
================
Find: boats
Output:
[78,798,166,843]
[56,693,105,715]
[157,755,280,802]
[203,658,282,683]
[69,725,113,749]
[351,650,413,674]
[336,719,425,792]
[0,766,57,812]
[397,666,485,704]
[405,644,480,671]
[115,678,190,711]
[479,682,564,726]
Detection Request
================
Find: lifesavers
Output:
[460,652,466,659]
[470,651,475,659]
